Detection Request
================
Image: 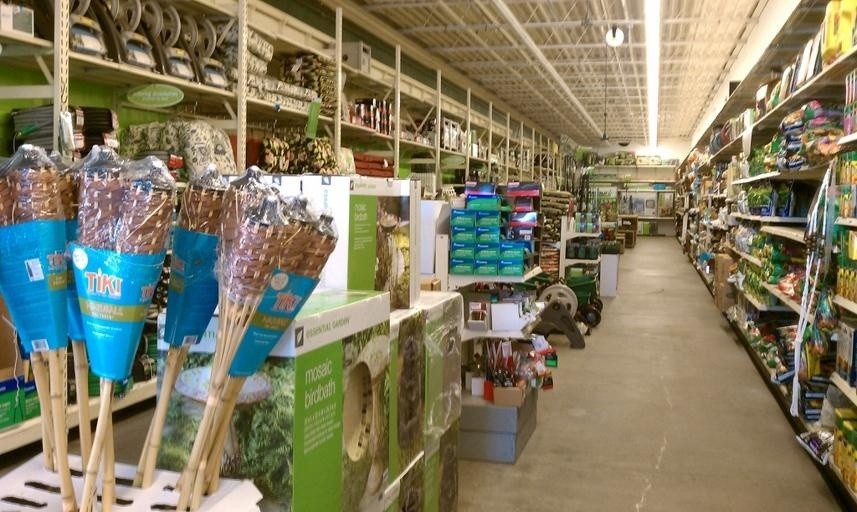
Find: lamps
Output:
[600,22,610,148]
[605,25,624,47]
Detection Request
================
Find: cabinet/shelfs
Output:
[1,0,568,455]
[555,216,618,298]
[675,0,857,512]
[590,165,676,236]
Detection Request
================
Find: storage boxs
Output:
[618,214,657,252]
[715,254,737,313]
